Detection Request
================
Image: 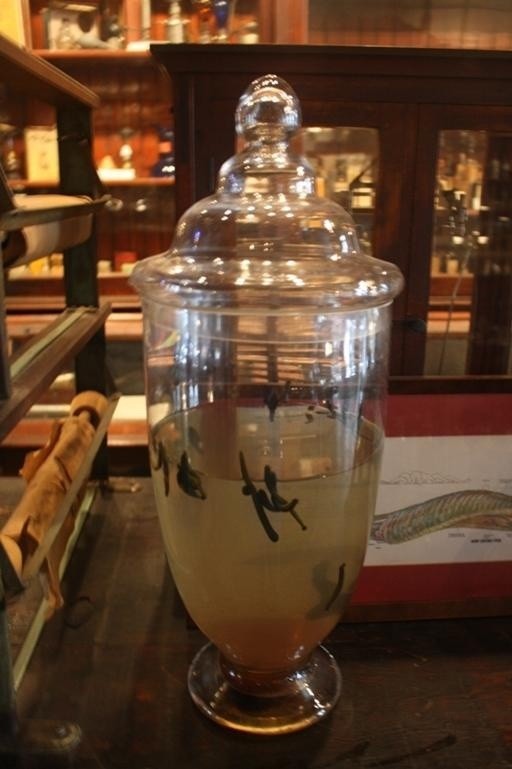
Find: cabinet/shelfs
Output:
[1,0,509,701]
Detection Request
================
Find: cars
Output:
[128,74,408,743]
